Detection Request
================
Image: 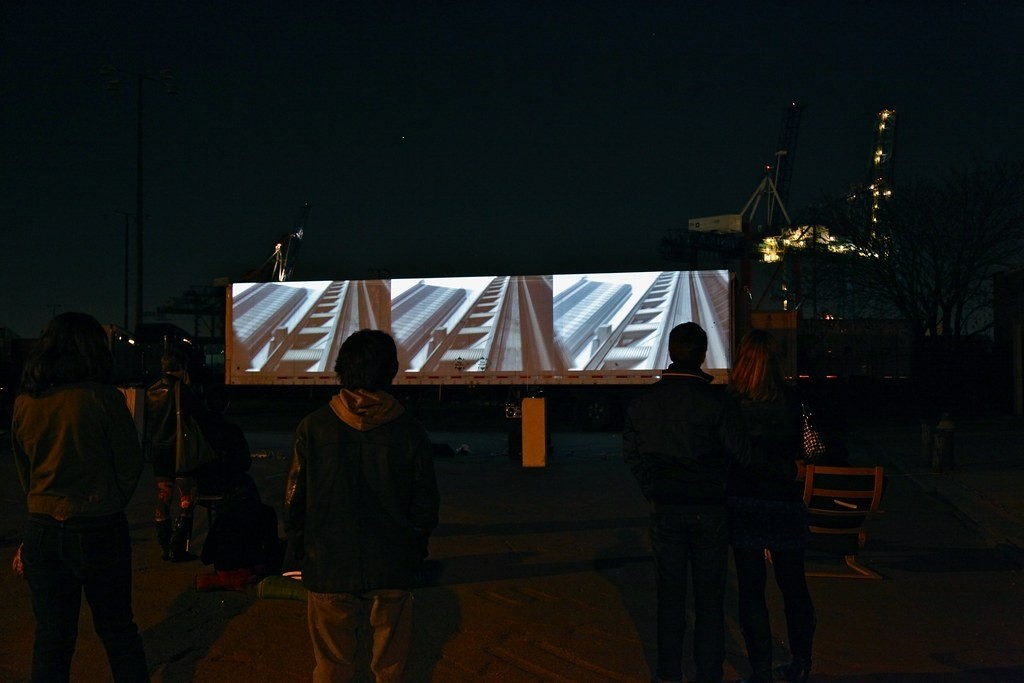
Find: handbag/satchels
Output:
[799,397,830,465]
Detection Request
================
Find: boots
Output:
[170,516,199,563]
[155,518,174,561]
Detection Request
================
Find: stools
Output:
[196,495,226,530]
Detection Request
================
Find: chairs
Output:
[801,465,885,581]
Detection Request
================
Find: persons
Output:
[12,311,151,683]
[146,349,263,562]
[622,323,817,683]
[283,330,440,683]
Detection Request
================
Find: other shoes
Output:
[773,662,809,683]
[733,672,773,683]
